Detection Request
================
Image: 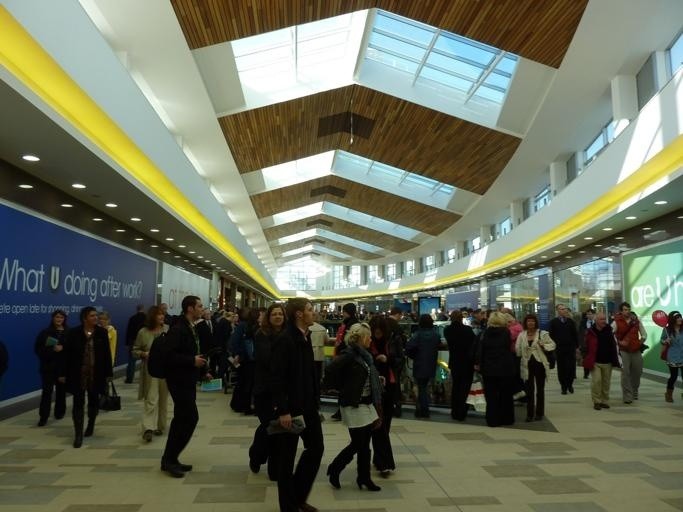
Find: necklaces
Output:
[88,332,92,335]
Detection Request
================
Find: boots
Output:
[356,448,381,492]
[326,451,353,489]
[664,388,674,404]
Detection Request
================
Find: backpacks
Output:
[146,326,182,380]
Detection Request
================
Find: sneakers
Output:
[594,403,610,411]
[160,455,193,479]
[331,413,342,421]
[143,429,163,443]
[295,501,320,512]
[381,468,390,478]
[561,384,575,395]
[524,414,544,423]
[249,458,261,474]
[37,413,94,448]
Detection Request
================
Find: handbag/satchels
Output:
[98,380,122,412]
[538,328,556,370]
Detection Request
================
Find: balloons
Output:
[652,310,668,327]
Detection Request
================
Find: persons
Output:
[660,311,683,402]
[125,296,647,511]
[58,306,113,448]
[99,311,118,367]
[0,342,9,377]
[34,309,70,427]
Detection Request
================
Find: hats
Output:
[343,303,356,317]
